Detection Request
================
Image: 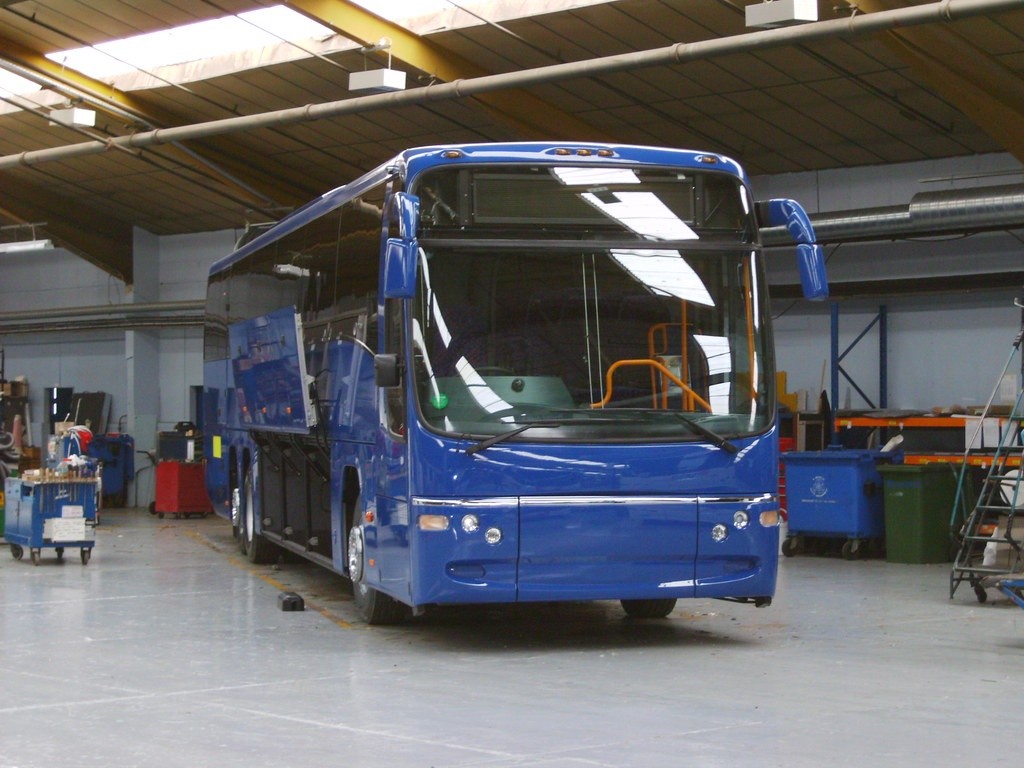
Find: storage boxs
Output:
[0,379,28,398]
[23,445,41,459]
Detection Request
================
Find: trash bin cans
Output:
[779,443,906,561]
[876,461,974,564]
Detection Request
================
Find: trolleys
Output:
[4,472,100,564]
[156,459,213,519]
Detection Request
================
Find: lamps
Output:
[348,49,407,95]
[744,0,819,29]
[49,107,96,127]
[0,222,57,254]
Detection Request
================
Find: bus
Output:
[199,135,827,627]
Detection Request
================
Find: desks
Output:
[834,416,1024,445]
[903,452,1024,540]
